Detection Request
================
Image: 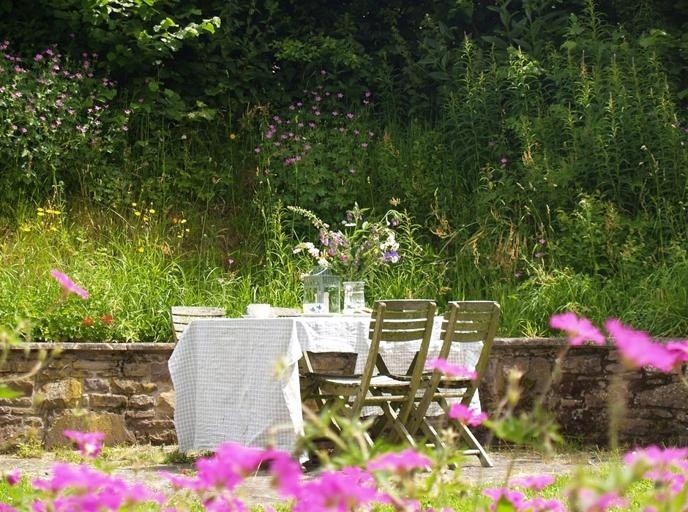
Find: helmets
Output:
[304,313,335,317]
[241,315,278,319]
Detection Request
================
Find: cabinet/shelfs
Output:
[314,292,330,313]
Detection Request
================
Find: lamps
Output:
[168,306,230,347]
[400,300,501,469]
[316,299,438,458]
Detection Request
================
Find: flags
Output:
[342,281,365,311]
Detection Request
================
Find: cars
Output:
[342,281,366,313]
[247,303,271,316]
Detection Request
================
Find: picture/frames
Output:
[188,314,479,473]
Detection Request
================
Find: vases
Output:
[278,190,417,280]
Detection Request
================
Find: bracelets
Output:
[303,304,325,314]
